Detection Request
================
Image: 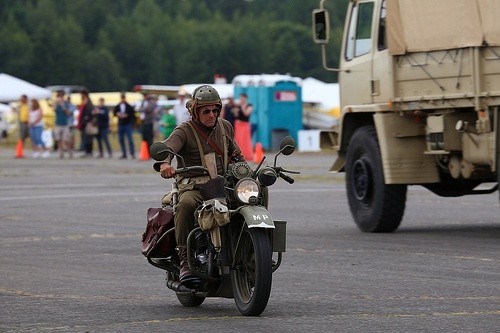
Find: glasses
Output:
[204,109,219,114]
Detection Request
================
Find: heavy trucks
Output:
[310,1,500,234]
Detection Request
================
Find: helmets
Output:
[191,85,221,104]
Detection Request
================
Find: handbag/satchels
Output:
[141,206,176,257]
[84,121,99,135]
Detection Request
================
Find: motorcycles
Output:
[140,134,302,318]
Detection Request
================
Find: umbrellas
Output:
[0,73,50,101]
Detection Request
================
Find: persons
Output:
[153,86,277,282]
[113,90,192,159]
[223,93,253,160]
[17,91,112,158]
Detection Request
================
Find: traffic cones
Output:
[139,140,150,160]
[253,142,264,163]
[15,139,24,158]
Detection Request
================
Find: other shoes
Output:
[272,257,276,266]
[176,248,192,281]
